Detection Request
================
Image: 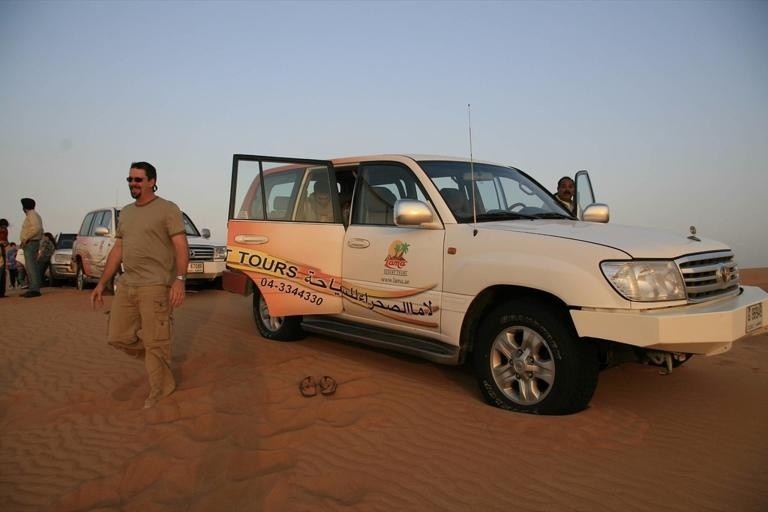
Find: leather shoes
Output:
[24,292,40,297]
[18,292,28,297]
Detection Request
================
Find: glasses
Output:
[126,178,143,183]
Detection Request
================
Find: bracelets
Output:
[175,275,186,280]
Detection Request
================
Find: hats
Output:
[20,198,34,207]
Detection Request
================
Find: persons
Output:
[20,198,42,298]
[304,181,332,222]
[90,162,189,408]
[6,241,17,287]
[542,177,582,219]
[38,232,57,280]
[0,219,8,297]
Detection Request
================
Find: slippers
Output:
[301,376,316,396]
[320,376,336,395]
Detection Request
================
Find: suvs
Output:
[13,233,77,287]
[70,207,227,294]
[220,103,768,415]
